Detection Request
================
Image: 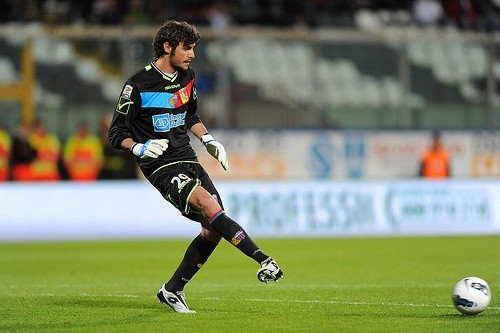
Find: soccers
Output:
[452,277,491,316]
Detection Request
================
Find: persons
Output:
[0,114,138,181]
[108,19,284,314]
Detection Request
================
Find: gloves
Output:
[200,133,229,170]
[131,138,169,158]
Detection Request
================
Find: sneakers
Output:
[157,283,196,314]
[257,256,284,283]
